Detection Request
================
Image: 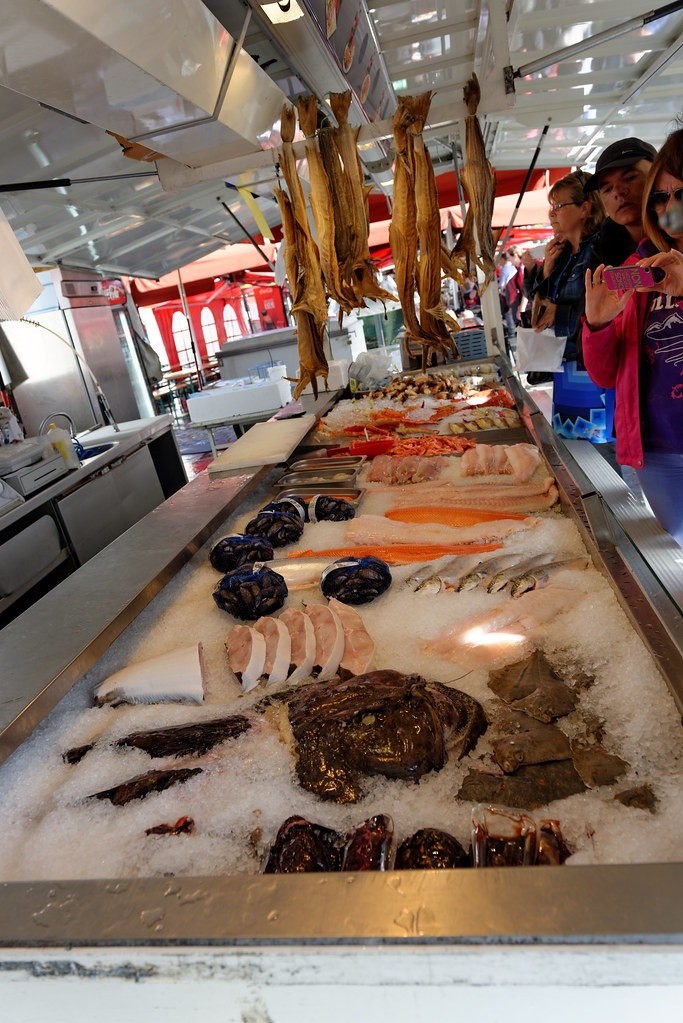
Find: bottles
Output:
[47,423,79,469]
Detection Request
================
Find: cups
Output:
[267,365,287,381]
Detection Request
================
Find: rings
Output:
[544,322,550,327]
[599,279,605,284]
[549,251,553,255]
[592,280,601,284]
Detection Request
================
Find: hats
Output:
[583,138,658,193]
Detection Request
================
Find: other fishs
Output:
[61,71,662,874]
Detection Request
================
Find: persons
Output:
[591,137,658,288]
[582,129,683,553]
[498,246,543,338]
[532,172,587,371]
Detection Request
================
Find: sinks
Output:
[76,441,120,466]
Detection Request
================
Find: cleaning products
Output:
[46,422,80,469]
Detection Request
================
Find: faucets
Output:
[38,410,77,438]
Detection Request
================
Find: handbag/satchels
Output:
[552,361,617,444]
[515,326,568,373]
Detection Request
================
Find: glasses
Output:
[550,201,576,213]
[649,187,683,210]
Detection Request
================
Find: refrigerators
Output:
[0,267,157,432]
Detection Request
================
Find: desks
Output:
[151,356,218,415]
[189,407,285,461]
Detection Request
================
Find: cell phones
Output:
[604,264,656,291]
[528,244,547,260]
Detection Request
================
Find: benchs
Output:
[152,370,219,414]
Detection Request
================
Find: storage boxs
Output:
[187,362,351,422]
